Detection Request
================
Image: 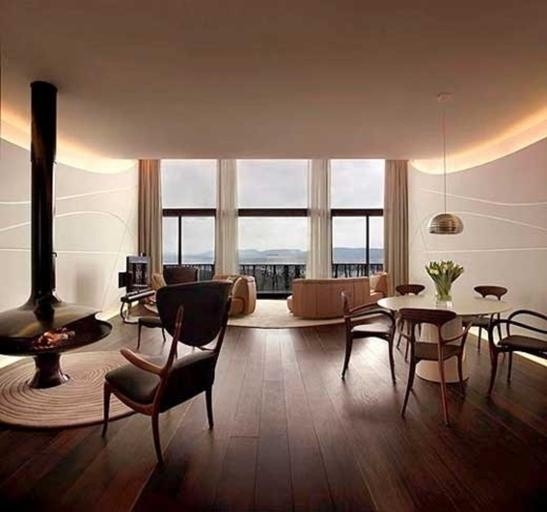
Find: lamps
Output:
[428,92,464,234]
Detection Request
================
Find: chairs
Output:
[485,307,547,396]
[463,285,506,358]
[397,305,467,427]
[99,278,237,464]
[395,284,425,360]
[338,288,402,383]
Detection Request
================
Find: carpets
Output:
[144,298,384,329]
[0,349,151,429]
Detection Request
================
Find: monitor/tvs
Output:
[126,255,150,297]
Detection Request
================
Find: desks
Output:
[376,295,512,384]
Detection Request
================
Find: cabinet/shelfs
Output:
[120,289,159,324]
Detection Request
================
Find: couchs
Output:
[287,270,393,319]
[212,271,257,317]
